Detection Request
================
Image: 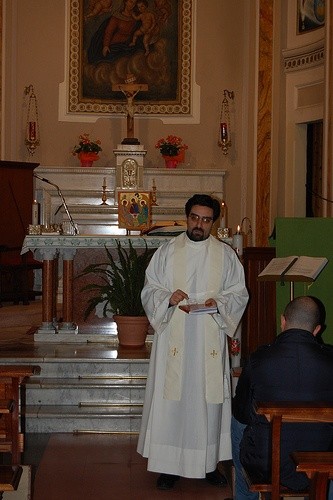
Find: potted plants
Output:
[73,238,160,346]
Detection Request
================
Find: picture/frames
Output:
[58,0,201,125]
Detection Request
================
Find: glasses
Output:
[188,215,214,224]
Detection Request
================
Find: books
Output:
[258,256,328,280]
[179,304,217,315]
[142,220,187,233]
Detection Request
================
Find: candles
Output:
[103,177,107,186]
[233,225,243,255]
[152,178,156,186]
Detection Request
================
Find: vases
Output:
[162,155,178,168]
[78,152,96,167]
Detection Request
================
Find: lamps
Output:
[24,84,42,155]
[217,88,234,154]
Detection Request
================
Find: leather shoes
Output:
[205,469,227,486]
[156,474,180,489]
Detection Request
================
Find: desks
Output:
[238,401,333,500]
[20,234,233,342]
[0,364,40,484]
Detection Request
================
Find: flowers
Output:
[155,135,189,155]
[69,133,103,157]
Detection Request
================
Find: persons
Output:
[118,86,141,131]
[230,296,333,500]
[137,194,249,490]
